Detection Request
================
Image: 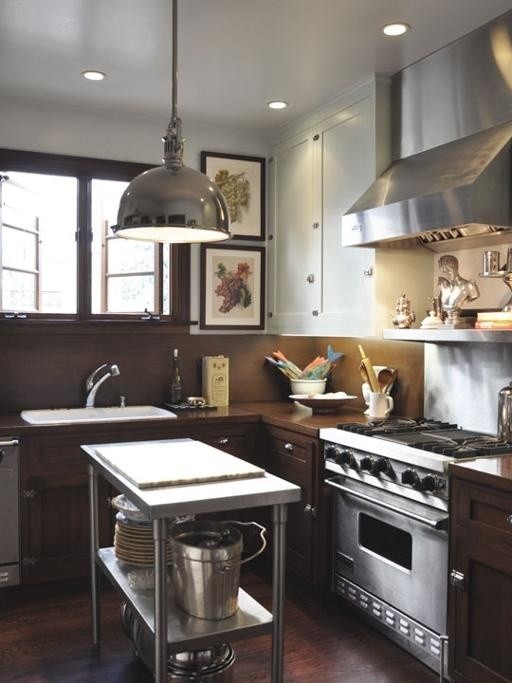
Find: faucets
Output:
[84,363,121,409]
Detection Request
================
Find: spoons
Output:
[378,368,392,392]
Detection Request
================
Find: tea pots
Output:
[495,382,511,441]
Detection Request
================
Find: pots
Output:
[167,640,236,683]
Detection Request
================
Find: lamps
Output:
[109,0,233,245]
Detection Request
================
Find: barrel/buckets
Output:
[170,519,268,623]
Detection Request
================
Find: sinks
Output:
[20,405,179,428]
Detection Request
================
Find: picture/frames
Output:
[200,151,266,241]
[200,243,265,330]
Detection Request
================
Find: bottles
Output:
[168,349,183,404]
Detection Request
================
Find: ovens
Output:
[318,470,448,681]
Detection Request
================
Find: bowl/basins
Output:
[289,377,327,394]
[111,492,153,524]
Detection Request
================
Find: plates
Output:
[114,512,174,568]
[288,393,357,413]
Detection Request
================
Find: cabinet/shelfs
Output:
[447,475,512,683]
[265,74,435,339]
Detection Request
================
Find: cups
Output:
[370,392,395,418]
[188,396,206,406]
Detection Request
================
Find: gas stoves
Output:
[322,415,512,510]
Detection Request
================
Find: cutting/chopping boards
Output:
[95,440,266,489]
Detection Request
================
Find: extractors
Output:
[341,8,512,249]
